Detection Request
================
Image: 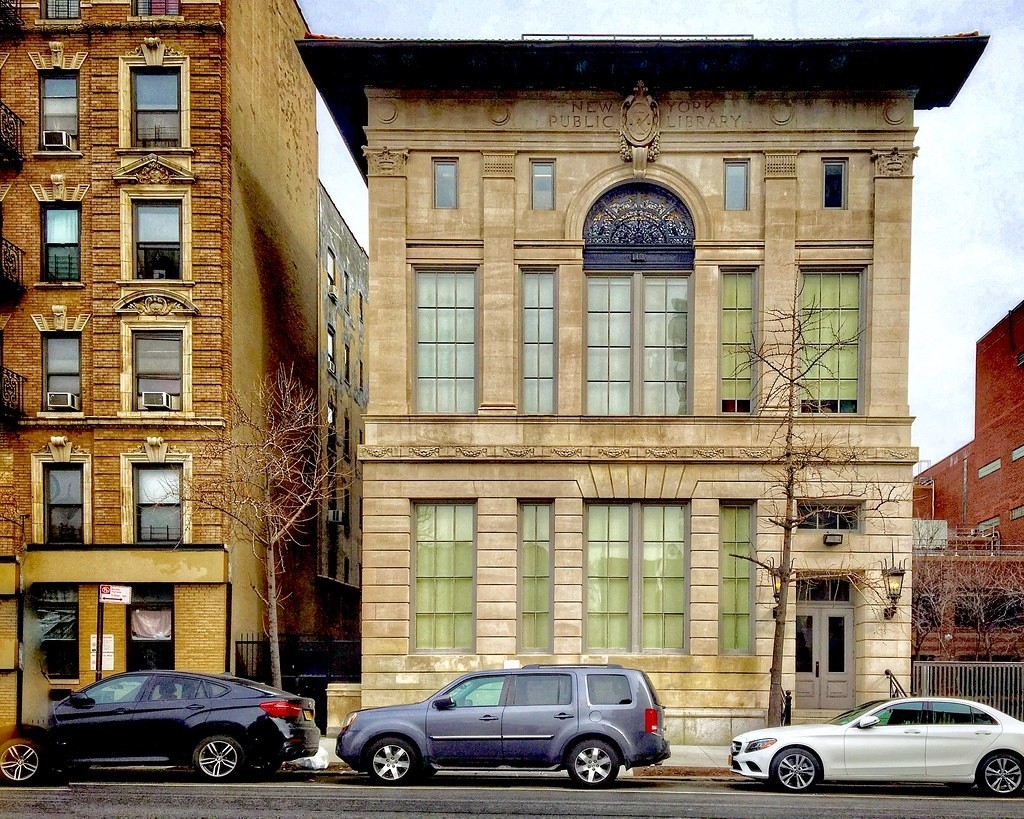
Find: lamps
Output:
[770,566,781,620]
[824,534,842,546]
[879,558,908,620]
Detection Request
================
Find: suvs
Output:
[334,663,671,786]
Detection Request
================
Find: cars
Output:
[0,668,322,786]
[728,697,1024,798]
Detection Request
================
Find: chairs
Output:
[158,684,177,700]
